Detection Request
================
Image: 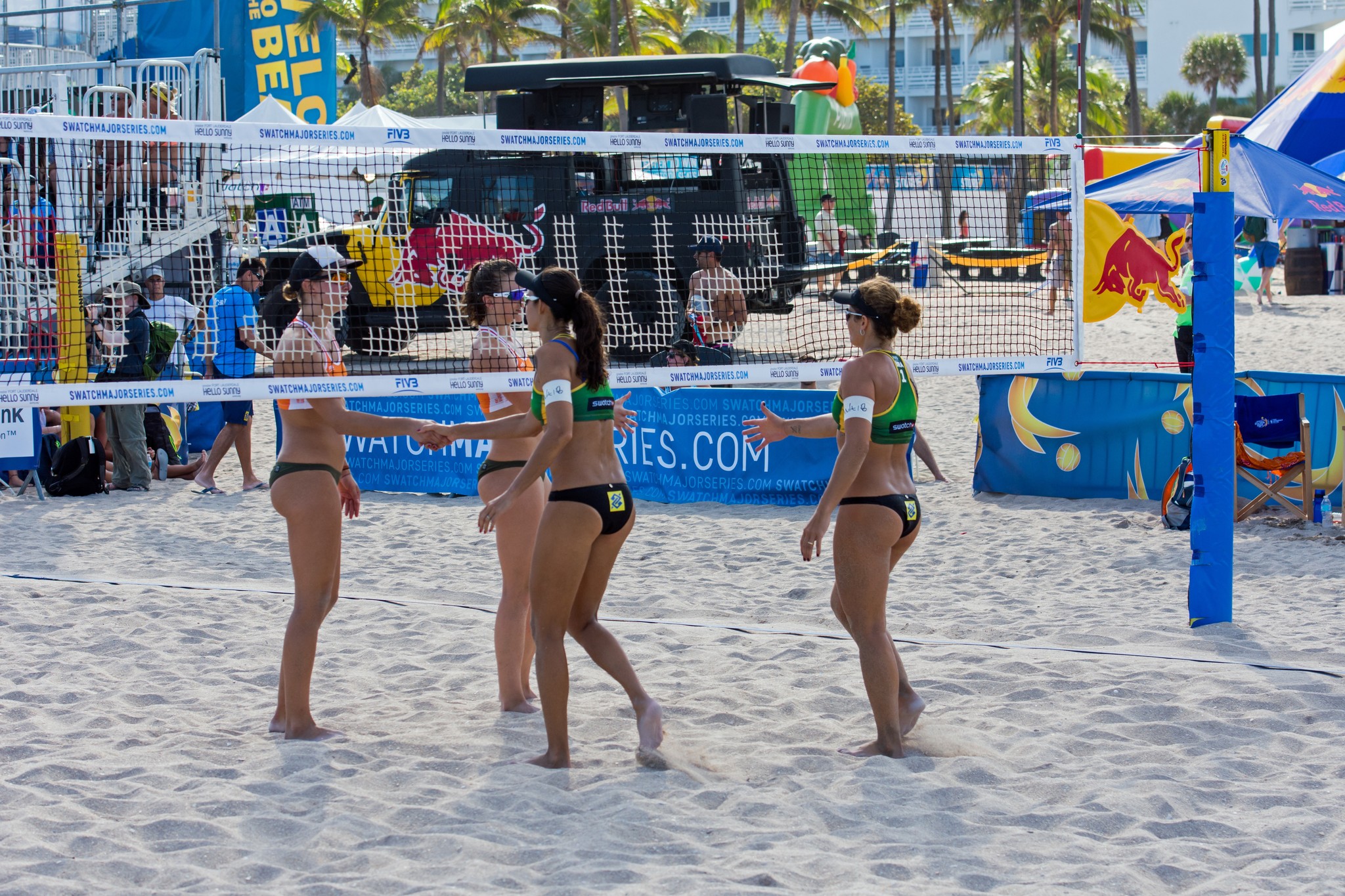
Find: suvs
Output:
[257,52,840,356]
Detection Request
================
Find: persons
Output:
[79,84,146,229]
[415,268,664,770]
[103,82,184,210]
[0,136,1280,494]
[268,243,452,741]
[741,280,922,760]
[459,258,638,715]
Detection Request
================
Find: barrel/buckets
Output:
[1285,248,1323,296]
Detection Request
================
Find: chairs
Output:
[650,346,731,389]
[1234,392,1313,523]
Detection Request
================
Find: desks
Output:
[844,248,910,281]
[964,247,1046,281]
[935,238,996,265]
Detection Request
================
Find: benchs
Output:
[9,181,183,306]
[858,246,1045,282]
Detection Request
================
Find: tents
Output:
[220,94,432,224]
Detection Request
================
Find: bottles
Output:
[147,454,152,469]
[1321,496,1333,528]
[828,246,840,257]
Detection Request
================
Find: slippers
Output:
[154,448,168,481]
[191,487,219,495]
[242,482,270,492]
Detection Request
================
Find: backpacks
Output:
[1161,455,1194,531]
[44,436,109,497]
[1242,216,1267,243]
[127,311,178,381]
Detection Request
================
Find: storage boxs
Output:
[254,193,319,253]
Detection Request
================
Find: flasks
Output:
[1314,494,1325,523]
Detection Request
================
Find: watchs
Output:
[186,333,193,341]
[714,317,719,326]
[91,320,100,327]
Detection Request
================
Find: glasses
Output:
[110,95,125,101]
[829,200,836,203]
[311,271,351,286]
[523,293,557,306]
[245,268,264,282]
[845,308,879,322]
[476,288,528,301]
[354,214,365,217]
[667,351,681,359]
[696,250,710,256]
[147,278,162,283]
[1184,236,1193,242]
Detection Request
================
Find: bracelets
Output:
[1047,261,1051,264]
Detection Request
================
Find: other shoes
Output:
[818,293,832,301]
[829,289,838,299]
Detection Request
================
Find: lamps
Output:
[362,173,377,183]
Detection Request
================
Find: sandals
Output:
[105,484,127,491]
[127,485,149,492]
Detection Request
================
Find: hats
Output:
[104,281,151,310]
[833,287,891,326]
[687,236,723,254]
[515,270,558,303]
[367,197,384,208]
[290,245,363,291]
[354,209,365,214]
[145,265,164,279]
[821,194,836,203]
[26,106,42,115]
[664,340,697,358]
